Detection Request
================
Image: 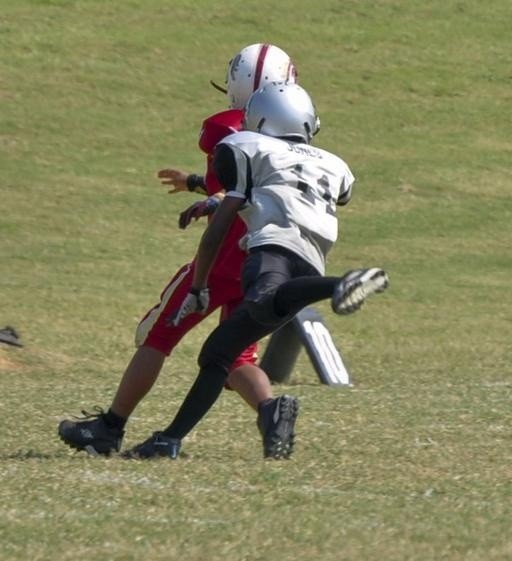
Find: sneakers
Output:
[59,406,124,457]
[256,395,300,460]
[330,267,387,314]
[124,431,181,459]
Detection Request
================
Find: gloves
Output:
[167,286,210,327]
[178,195,220,230]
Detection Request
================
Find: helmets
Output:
[245,81,321,144]
[226,44,296,109]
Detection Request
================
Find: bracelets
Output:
[187,174,207,195]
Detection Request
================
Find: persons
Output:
[114,79,391,467]
[55,42,302,464]
